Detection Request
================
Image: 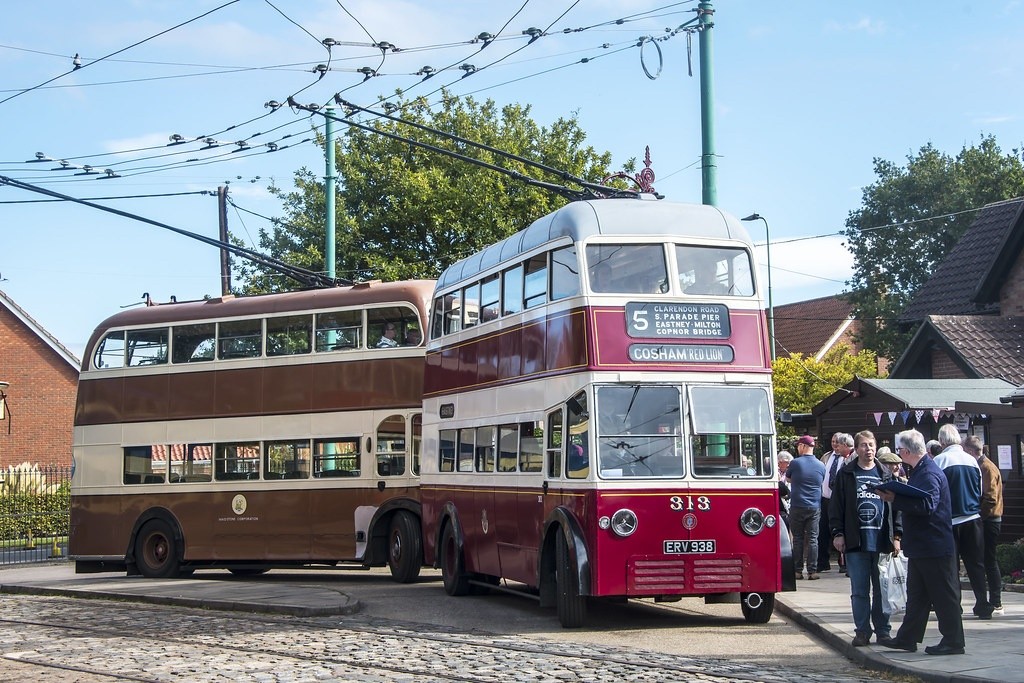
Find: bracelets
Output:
[895,538,901,540]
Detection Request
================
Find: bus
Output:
[420,199,783,627]
[68,278,438,578]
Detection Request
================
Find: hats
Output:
[878,452,902,463]
[795,435,815,447]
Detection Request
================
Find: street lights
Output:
[741,214,776,363]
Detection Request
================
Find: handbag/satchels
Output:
[877,550,908,616]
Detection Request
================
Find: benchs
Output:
[123,468,358,478]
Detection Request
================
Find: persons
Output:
[566,398,672,477]
[589,258,733,295]
[829,431,904,645]
[740,424,1004,620]
[401,329,420,347]
[375,321,398,348]
[881,431,966,653]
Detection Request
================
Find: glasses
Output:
[386,327,395,331]
[896,448,910,453]
[412,334,420,338]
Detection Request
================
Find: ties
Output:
[828,454,841,491]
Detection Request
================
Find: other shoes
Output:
[807,572,820,580]
[979,614,992,619]
[991,605,1004,613]
[845,574,850,578]
[795,572,805,579]
[851,629,872,647]
[839,567,847,573]
[818,564,831,571]
[876,634,892,646]
[887,637,917,652]
[925,640,965,655]
